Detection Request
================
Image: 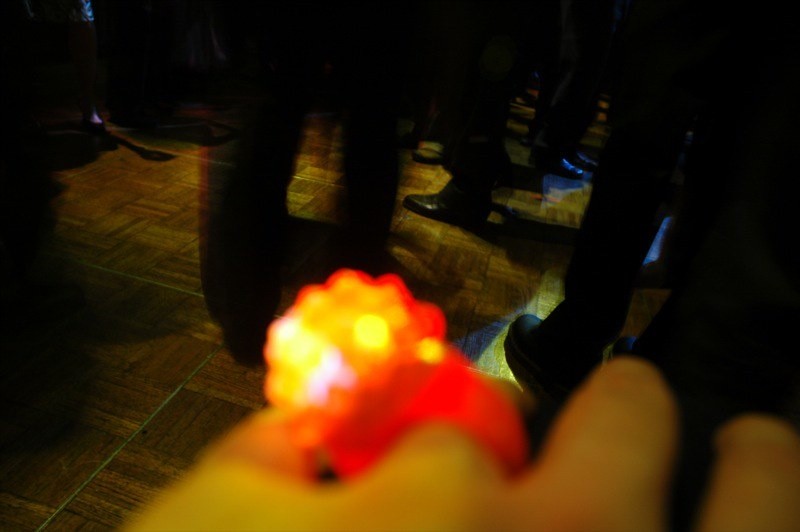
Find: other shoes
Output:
[614,336,639,357]
[532,160,584,181]
[568,150,598,173]
[82,110,103,129]
[504,314,572,414]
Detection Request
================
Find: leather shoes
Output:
[403,192,487,232]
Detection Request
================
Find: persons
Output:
[0,0,800,471]
[124,356,800,532]
